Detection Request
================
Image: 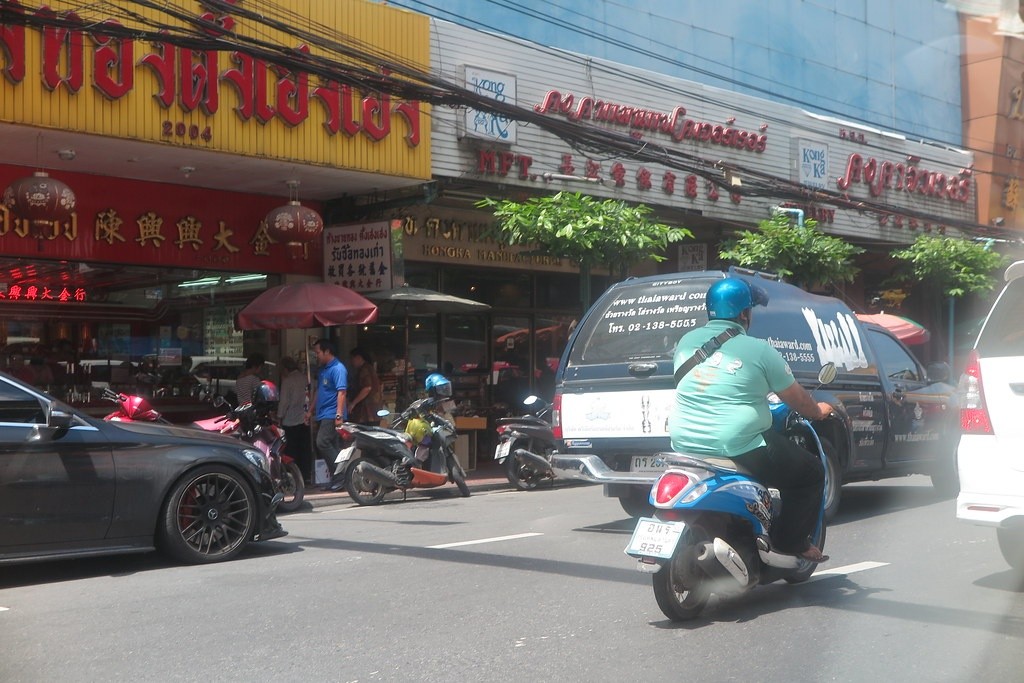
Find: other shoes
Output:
[331,478,345,491]
[320,483,331,491]
[767,533,826,561]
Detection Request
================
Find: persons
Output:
[236,347,383,479]
[536,357,553,384]
[669,278,832,563]
[304,338,348,490]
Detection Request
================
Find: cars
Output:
[191,356,277,404]
[0,372,290,564]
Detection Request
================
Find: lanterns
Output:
[3,171,77,236]
[264,200,323,258]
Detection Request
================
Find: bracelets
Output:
[335,415,344,420]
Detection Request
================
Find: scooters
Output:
[212,381,305,512]
[623,360,838,623]
[492,392,556,491]
[332,373,471,507]
[99,387,239,434]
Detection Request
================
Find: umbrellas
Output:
[361,283,491,408]
[234,282,378,453]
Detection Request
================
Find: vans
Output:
[954,260,1023,570]
[549,265,959,517]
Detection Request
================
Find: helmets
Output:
[425,373,449,391]
[252,381,280,409]
[705,277,769,321]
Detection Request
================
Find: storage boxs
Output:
[454,416,487,427]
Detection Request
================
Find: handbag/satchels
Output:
[404,413,433,446]
